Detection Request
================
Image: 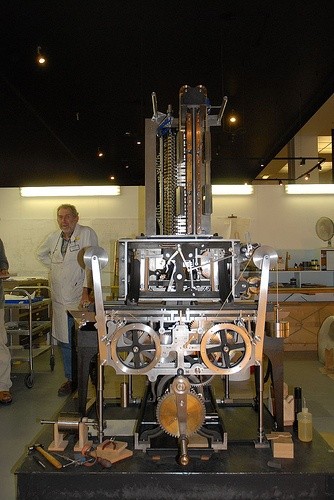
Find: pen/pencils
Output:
[34,456,46,469]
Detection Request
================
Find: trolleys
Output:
[4,286,54,390]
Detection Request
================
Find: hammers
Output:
[28,443,62,469]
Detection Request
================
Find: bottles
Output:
[298,406,313,442]
[277,256,320,271]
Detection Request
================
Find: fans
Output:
[315,216,334,249]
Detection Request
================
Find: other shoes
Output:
[0,389,12,403]
[57,381,75,397]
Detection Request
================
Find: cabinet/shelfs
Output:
[240,250,334,295]
[2,279,50,345]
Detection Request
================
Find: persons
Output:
[0,238,12,402]
[35,204,98,396]
